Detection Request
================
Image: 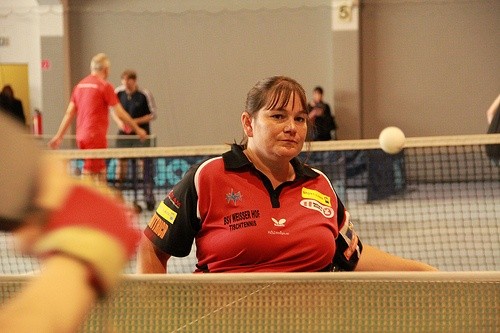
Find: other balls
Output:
[379,126,405,154]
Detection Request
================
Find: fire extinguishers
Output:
[33,109,42,137]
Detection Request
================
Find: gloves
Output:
[30,173,139,300]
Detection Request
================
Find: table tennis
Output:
[122,121,134,133]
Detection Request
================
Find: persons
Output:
[114,70,157,215]
[304,86,337,142]
[483,94,500,168]
[0,112,142,333]
[136,74,439,275]
[47,52,147,185]
